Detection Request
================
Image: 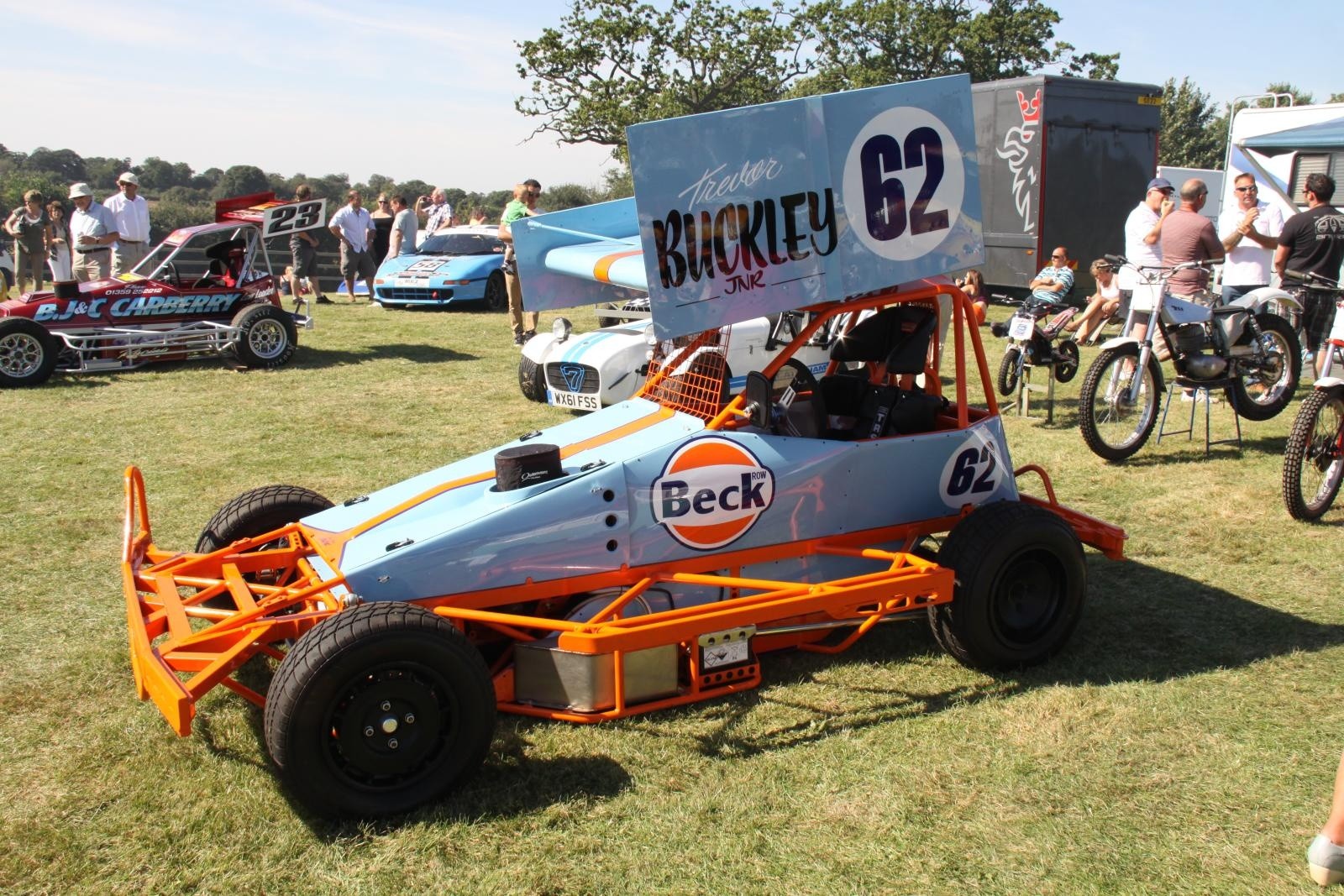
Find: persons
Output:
[1274,173,1344,376]
[1119,178,1175,380]
[370,192,393,218]
[43,200,71,281]
[470,206,488,224]
[950,269,989,325]
[1160,178,1226,404]
[67,183,120,283]
[328,190,377,302]
[280,266,293,295]
[415,187,453,240]
[103,172,151,276]
[1218,173,1284,306]
[5,189,53,295]
[289,184,334,305]
[367,195,418,307]
[497,179,547,347]
[990,247,1074,337]
[1064,259,1119,346]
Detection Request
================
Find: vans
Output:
[1155,165,1224,229]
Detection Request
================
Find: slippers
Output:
[1074,338,1087,345]
[1063,324,1077,332]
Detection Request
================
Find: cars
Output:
[0,192,328,389]
[119,274,1134,823]
[593,297,652,328]
[516,305,927,411]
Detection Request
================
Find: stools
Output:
[1157,375,1242,457]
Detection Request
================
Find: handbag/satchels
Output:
[13,207,27,235]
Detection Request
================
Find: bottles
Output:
[422,196,432,202]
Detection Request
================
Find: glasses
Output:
[1151,189,1171,196]
[529,191,540,197]
[378,200,389,204]
[73,196,84,200]
[1236,185,1256,191]
[120,181,133,186]
[1051,255,1064,259]
[1302,189,1311,194]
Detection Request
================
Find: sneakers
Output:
[524,330,537,343]
[515,334,524,346]
[1182,390,1220,404]
[501,262,514,275]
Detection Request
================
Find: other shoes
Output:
[991,322,1003,338]
[316,295,335,303]
[293,297,308,304]
[1245,382,1267,396]
[1118,368,1137,382]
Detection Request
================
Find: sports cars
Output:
[372,224,517,313]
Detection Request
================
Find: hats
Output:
[1147,179,1175,191]
[116,172,140,188]
[68,183,94,199]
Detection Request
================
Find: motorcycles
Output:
[989,293,1082,396]
[1077,253,1305,461]
[1281,267,1344,523]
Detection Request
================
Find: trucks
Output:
[1217,91,1344,285]
[942,71,1165,308]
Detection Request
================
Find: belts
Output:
[77,247,109,254]
[119,239,142,244]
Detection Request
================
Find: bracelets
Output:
[95,237,99,244]
